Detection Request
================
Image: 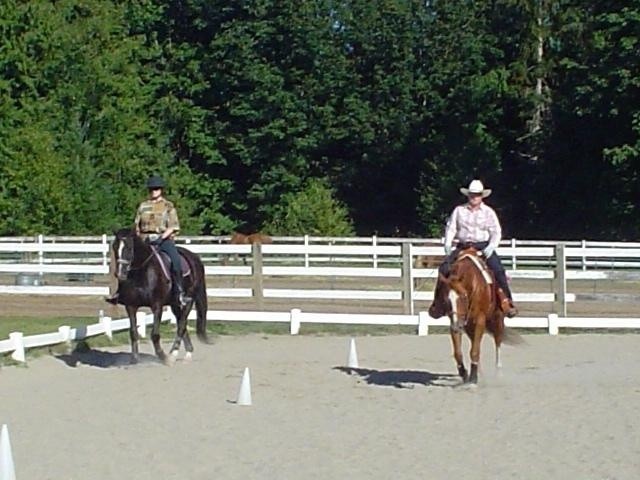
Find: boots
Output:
[171,270,193,304]
[106,294,125,305]
[495,286,518,317]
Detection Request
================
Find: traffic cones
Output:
[346,338,359,375]
[238,366,252,408]
[0,424,17,480]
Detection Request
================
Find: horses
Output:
[223,230,273,265]
[112,223,208,366]
[429,247,524,383]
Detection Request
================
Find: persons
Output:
[427,177,518,319]
[104,177,188,309]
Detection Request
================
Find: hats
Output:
[459,179,492,198]
[146,176,167,188]
